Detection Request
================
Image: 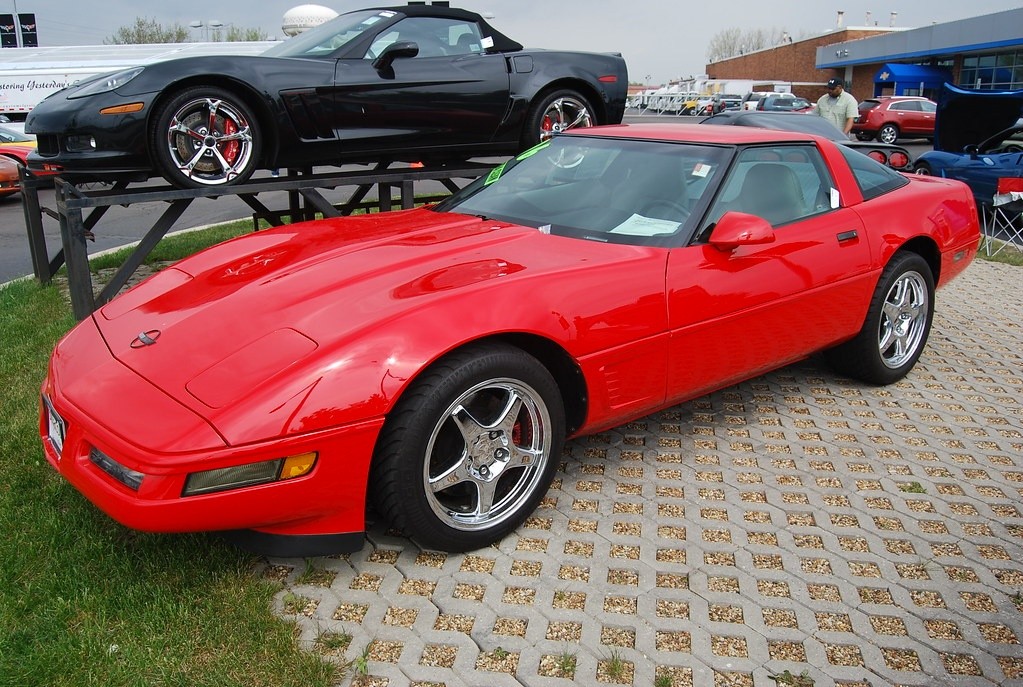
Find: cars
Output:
[850,95,937,144]
[0,129,63,200]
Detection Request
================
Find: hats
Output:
[825,77,844,90]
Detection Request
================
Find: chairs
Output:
[397,31,478,57]
[611,153,690,222]
[978,177,1023,258]
[722,164,810,227]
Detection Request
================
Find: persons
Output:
[812,76,859,137]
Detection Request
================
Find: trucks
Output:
[631,78,753,113]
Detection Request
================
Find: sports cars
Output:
[24,6,629,190]
[699,112,914,172]
[912,80,1023,219]
[38,123,981,558]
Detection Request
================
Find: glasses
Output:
[828,84,841,90]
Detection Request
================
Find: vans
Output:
[681,93,812,117]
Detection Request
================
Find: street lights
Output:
[189,21,223,42]
